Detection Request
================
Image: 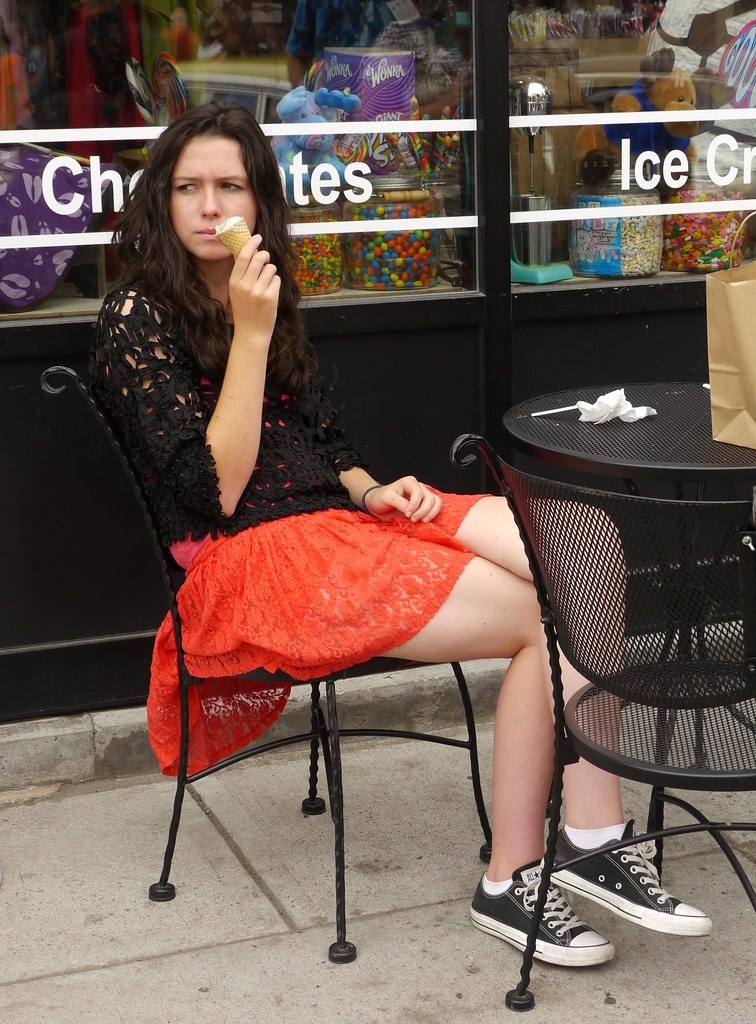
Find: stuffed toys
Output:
[572,46,701,182]
[269,87,363,207]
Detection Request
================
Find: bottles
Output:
[286,172,460,296]
[659,163,748,269]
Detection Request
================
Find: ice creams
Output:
[214,216,258,261]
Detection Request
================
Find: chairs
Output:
[39,361,492,963]
[450,433,756,1015]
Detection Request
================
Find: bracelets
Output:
[361,484,383,514]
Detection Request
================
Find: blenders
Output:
[464,74,571,283]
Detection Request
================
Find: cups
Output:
[516,192,554,266]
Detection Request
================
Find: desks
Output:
[503,380,756,923]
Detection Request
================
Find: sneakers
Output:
[469,860,615,966]
[540,818,712,936]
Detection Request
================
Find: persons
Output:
[82,99,714,968]
[283,0,459,91]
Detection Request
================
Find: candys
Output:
[125,52,188,125]
[586,187,751,277]
[286,61,464,294]
[49,148,91,165]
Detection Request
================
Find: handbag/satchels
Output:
[706,209,756,449]
